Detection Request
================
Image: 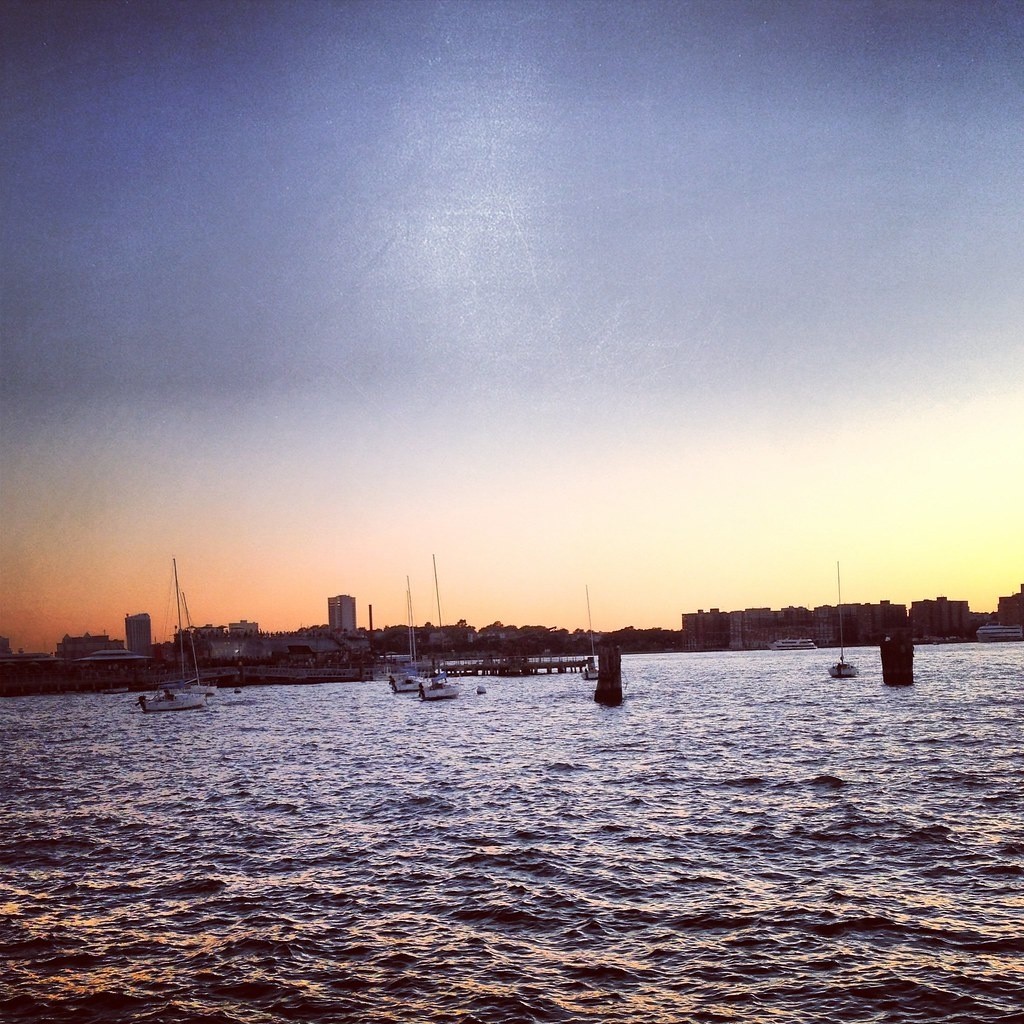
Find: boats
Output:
[976,621,1023,642]
[766,637,818,650]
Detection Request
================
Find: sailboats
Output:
[581,584,600,681]
[179,595,218,695]
[418,554,460,700]
[389,575,426,693]
[827,561,859,677]
[135,558,206,712]
